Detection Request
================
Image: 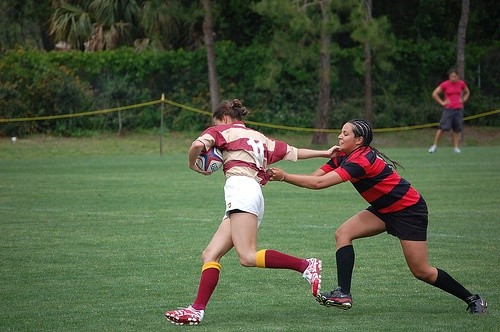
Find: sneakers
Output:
[164,307,206,325]
[315,287,352,311]
[466,294,488,314]
[302,257,321,297]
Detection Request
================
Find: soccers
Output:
[195,147,223,172]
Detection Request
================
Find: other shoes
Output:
[428,146,436,153]
[452,148,461,153]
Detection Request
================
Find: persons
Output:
[166,98,343,326]
[269,118,488,315]
[428,69,470,153]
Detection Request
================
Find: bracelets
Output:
[281,171,286,182]
